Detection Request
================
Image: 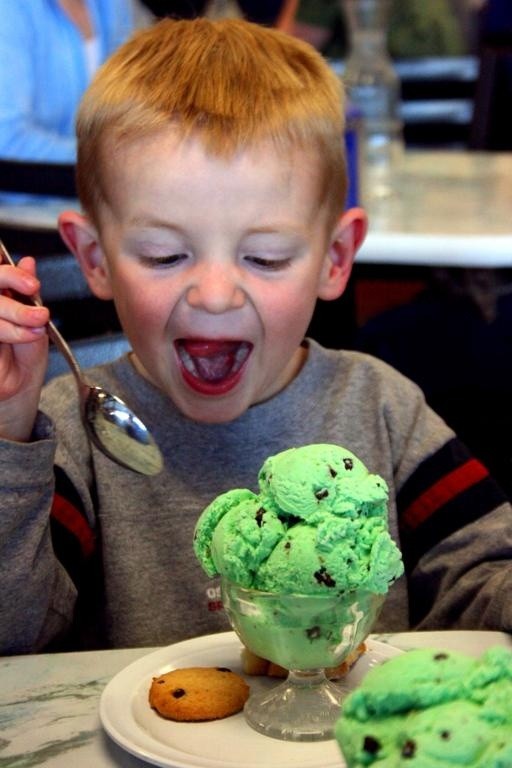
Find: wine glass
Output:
[217,565,388,742]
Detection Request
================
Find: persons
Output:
[0,0,155,343]
[144,0,350,60]
[452,0,509,153]
[0,22,512,658]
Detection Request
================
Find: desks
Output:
[2,146,512,349]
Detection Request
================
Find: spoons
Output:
[1,231,162,478]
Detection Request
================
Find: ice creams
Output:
[192,442,408,741]
[332,645,512,768]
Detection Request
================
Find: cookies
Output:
[148,666,250,721]
[240,644,367,680]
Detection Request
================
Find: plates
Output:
[98,630,409,767]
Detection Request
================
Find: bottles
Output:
[341,0,408,208]
